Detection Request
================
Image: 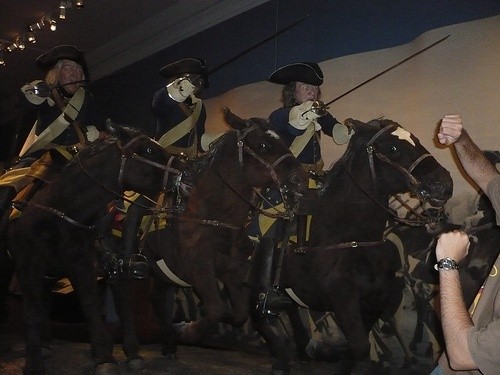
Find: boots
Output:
[121,197,152,273]
[254,236,292,305]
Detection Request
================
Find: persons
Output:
[435,115,500,375]
[233,61,351,323]
[0,45,121,286]
[122,56,210,283]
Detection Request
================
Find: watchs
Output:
[434,257,459,271]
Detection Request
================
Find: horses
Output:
[104,107,309,375]
[0,118,199,375]
[250,118,453,375]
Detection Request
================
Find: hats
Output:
[160,57,210,89]
[34,45,88,72]
[267,62,323,86]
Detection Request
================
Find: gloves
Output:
[288,101,327,130]
[21,80,55,107]
[166,73,203,103]
[332,123,355,145]
